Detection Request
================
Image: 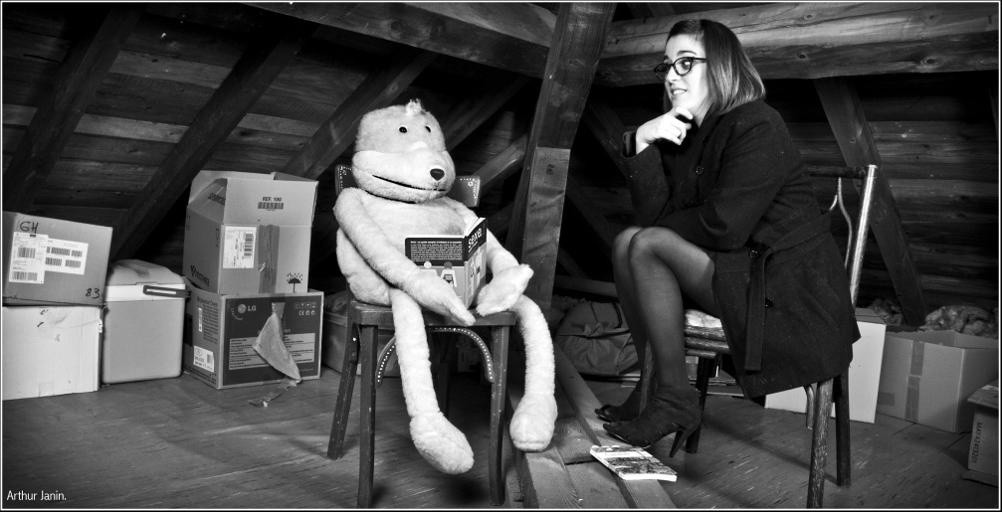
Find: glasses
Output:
[653,55,705,78]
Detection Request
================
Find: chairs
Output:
[669,164,877,509]
[326,167,548,508]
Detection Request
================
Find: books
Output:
[587,442,678,483]
[405,216,488,311]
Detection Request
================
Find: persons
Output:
[591,17,861,457]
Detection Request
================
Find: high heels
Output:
[595,382,703,457]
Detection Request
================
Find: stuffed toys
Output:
[337,99,558,478]
